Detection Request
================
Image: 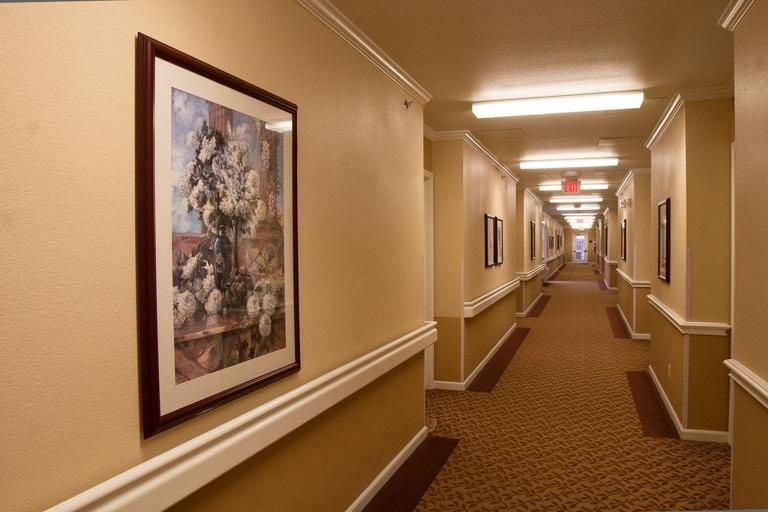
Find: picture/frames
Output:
[657,197,672,285]
[134,31,303,438]
[484,214,505,267]
